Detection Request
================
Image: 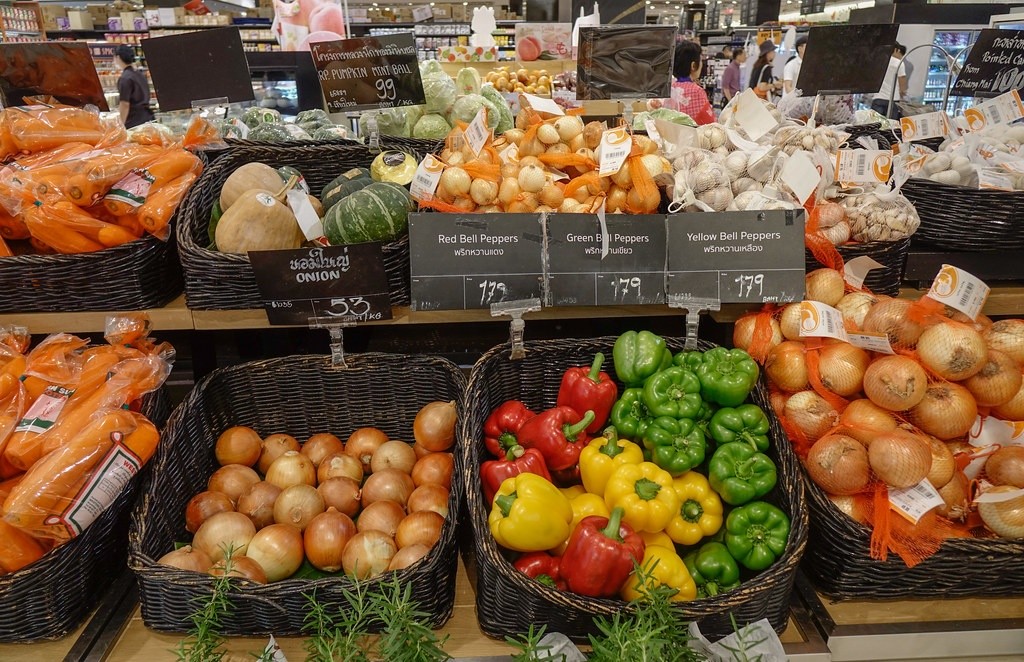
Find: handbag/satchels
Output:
[753,64,771,100]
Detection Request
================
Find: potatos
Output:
[485,68,577,95]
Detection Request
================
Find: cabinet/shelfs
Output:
[0,1,46,45]
[347,17,527,61]
[45,24,281,107]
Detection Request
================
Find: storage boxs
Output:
[576,24,679,100]
[184,0,275,26]
[67,12,94,30]
[121,9,144,31]
[87,3,110,25]
[159,7,184,26]
[366,3,524,24]
[143,4,164,25]
[41,6,66,27]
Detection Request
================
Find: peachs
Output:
[517,35,542,61]
[284,0,347,51]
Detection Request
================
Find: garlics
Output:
[658,120,1024,245]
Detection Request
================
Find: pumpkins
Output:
[213,150,417,256]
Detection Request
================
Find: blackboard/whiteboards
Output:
[408,212,544,312]
[0,42,110,112]
[308,33,426,114]
[544,213,666,306]
[796,22,901,97]
[667,208,806,304]
[139,26,256,114]
[949,27,1024,101]
[248,241,393,326]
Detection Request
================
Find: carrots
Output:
[0,351,159,576]
[0,103,201,258]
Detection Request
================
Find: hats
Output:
[115,44,135,64]
[759,40,778,58]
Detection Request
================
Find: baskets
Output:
[0,123,1024,649]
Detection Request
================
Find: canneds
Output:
[1,6,38,31]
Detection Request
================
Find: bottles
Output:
[922,77,973,113]
[699,73,718,84]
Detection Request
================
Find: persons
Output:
[722,46,732,59]
[783,36,808,96]
[111,44,154,128]
[663,43,715,125]
[899,46,913,89]
[721,48,745,106]
[749,40,783,100]
[871,41,906,117]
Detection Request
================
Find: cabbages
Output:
[358,59,513,140]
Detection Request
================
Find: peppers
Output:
[481,330,790,607]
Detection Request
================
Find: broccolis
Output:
[218,108,359,143]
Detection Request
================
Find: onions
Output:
[733,268,1023,542]
[431,114,671,213]
[155,400,458,583]
